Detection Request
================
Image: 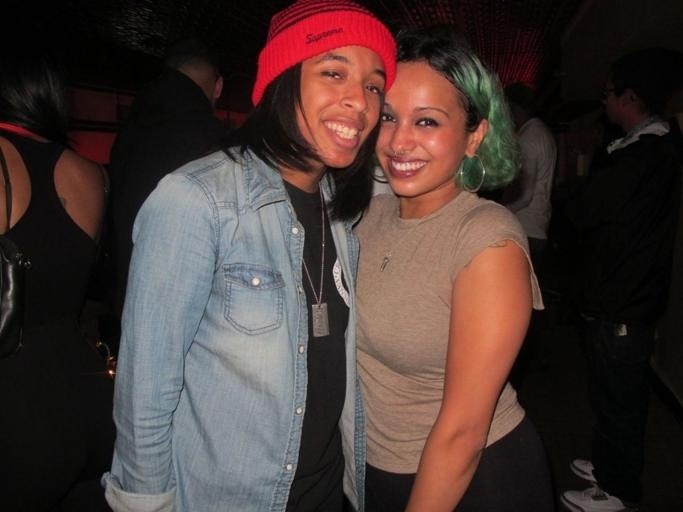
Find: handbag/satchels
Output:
[0,236,32,361]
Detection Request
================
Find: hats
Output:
[251,0,396,108]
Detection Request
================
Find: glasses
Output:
[602,86,619,98]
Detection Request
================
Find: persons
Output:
[2,2,682,509]
